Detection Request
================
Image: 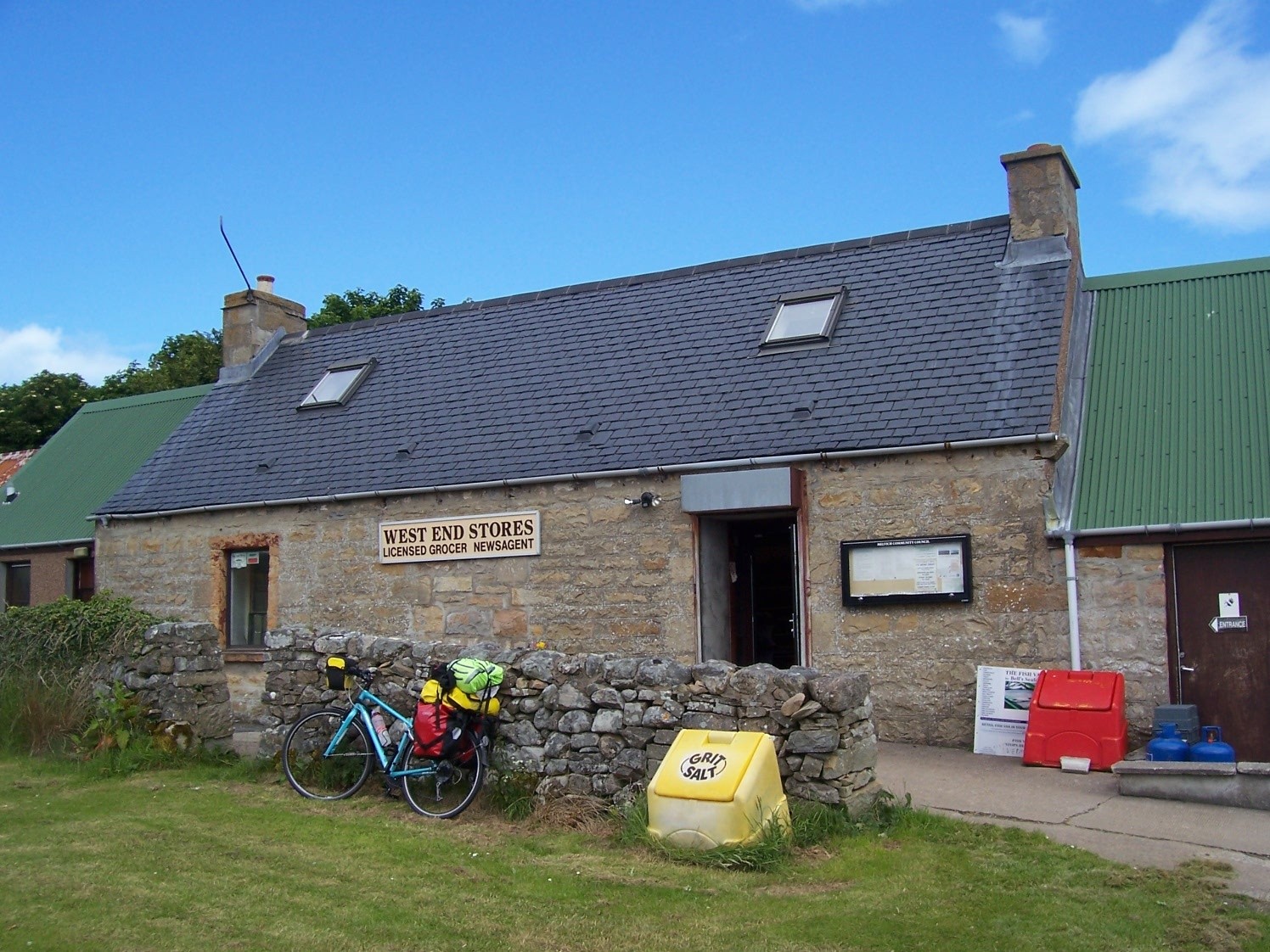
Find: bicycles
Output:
[281,665,490,819]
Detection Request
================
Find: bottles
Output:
[372,710,391,746]
